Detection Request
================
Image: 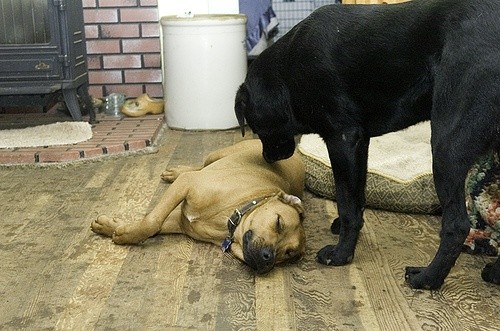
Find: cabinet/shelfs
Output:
[0,0,96,121]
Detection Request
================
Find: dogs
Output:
[89,138,306,275]
[234,0,500,292]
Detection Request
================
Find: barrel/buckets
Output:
[161,13,252,131]
